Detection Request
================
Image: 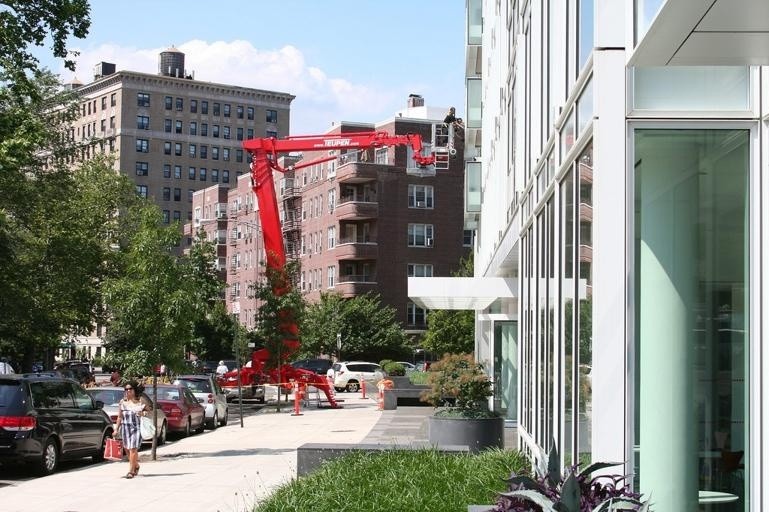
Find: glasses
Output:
[125,387,134,391]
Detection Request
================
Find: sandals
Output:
[125,464,140,479]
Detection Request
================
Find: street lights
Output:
[197,215,263,236]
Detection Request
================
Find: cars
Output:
[285,356,439,395]
[0,358,230,477]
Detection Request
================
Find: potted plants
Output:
[382,361,411,386]
[417,351,505,454]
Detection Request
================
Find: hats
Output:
[218,360,225,365]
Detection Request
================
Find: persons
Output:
[109,380,151,480]
[215,359,229,375]
[441,106,465,148]
[456,118,464,125]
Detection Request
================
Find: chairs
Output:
[714,447,744,490]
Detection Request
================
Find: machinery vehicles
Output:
[216,120,459,413]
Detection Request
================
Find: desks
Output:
[698,488,740,512]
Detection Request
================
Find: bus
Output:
[693,313,747,421]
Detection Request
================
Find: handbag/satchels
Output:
[104,436,124,461]
[139,415,157,440]
